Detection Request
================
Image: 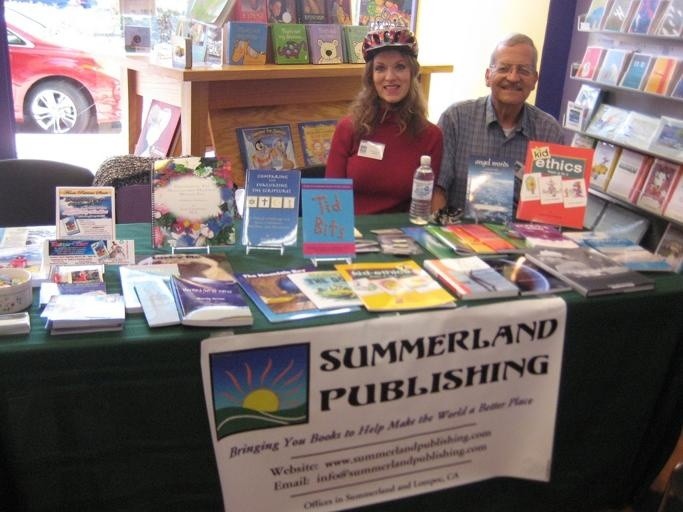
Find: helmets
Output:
[360,19,420,62]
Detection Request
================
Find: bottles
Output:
[408,154,436,227]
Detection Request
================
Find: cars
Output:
[5,8,121,133]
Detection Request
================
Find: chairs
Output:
[2,155,154,226]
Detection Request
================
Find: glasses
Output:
[491,61,538,78]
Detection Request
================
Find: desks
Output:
[0,217,682,512]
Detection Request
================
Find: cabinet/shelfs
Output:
[562,1,683,270]
